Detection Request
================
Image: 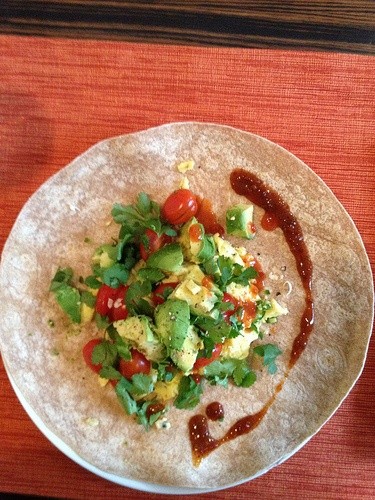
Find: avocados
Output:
[170,280,216,317]
[146,244,186,275]
[225,204,255,239]
[152,298,193,350]
[112,316,163,359]
[166,327,199,372]
[177,216,206,263]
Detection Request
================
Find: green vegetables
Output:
[46,193,281,429]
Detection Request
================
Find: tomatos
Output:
[94,284,131,320]
[139,227,175,261]
[118,350,151,379]
[160,189,198,225]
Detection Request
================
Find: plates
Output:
[0,120,374,495]
